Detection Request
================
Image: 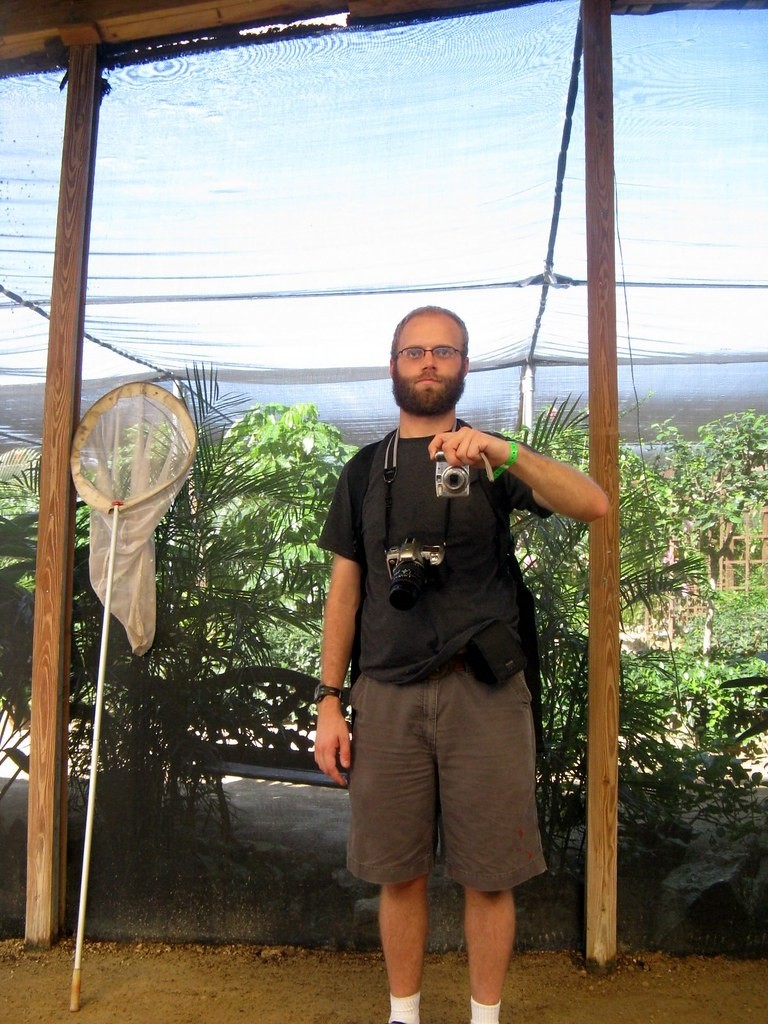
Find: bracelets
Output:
[493,441,518,479]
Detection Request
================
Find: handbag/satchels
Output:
[465,620,527,687]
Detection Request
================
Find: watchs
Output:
[314,683,344,703]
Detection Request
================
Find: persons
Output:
[313,307,610,1023]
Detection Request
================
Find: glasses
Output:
[395,345,463,361]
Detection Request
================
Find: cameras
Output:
[385,537,448,611]
[435,452,469,497]
